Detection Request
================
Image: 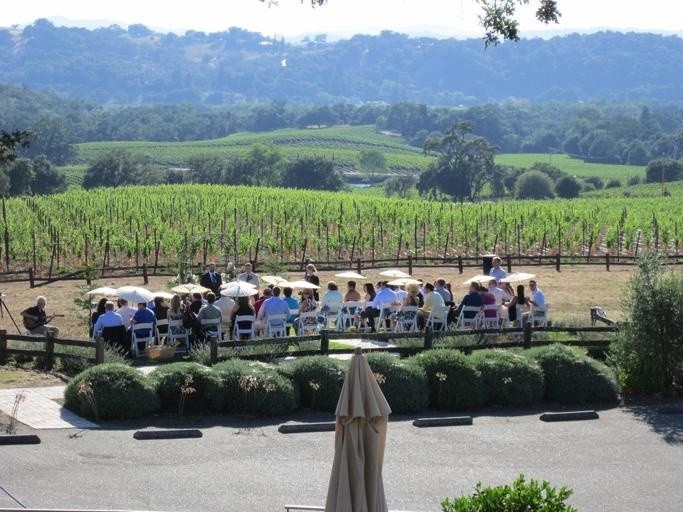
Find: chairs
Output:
[263,300,451,341]
[455,303,548,330]
[93,310,255,357]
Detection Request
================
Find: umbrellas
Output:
[323,347,393,512]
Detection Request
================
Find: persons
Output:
[488,258,505,284]
[345,280,546,330]
[20,296,59,338]
[199,263,222,295]
[215,281,341,339]
[305,263,319,301]
[88,291,222,352]
[239,263,259,288]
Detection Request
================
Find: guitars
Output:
[22,314,64,328]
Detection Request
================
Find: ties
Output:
[212,274,216,283]
[247,274,250,282]
